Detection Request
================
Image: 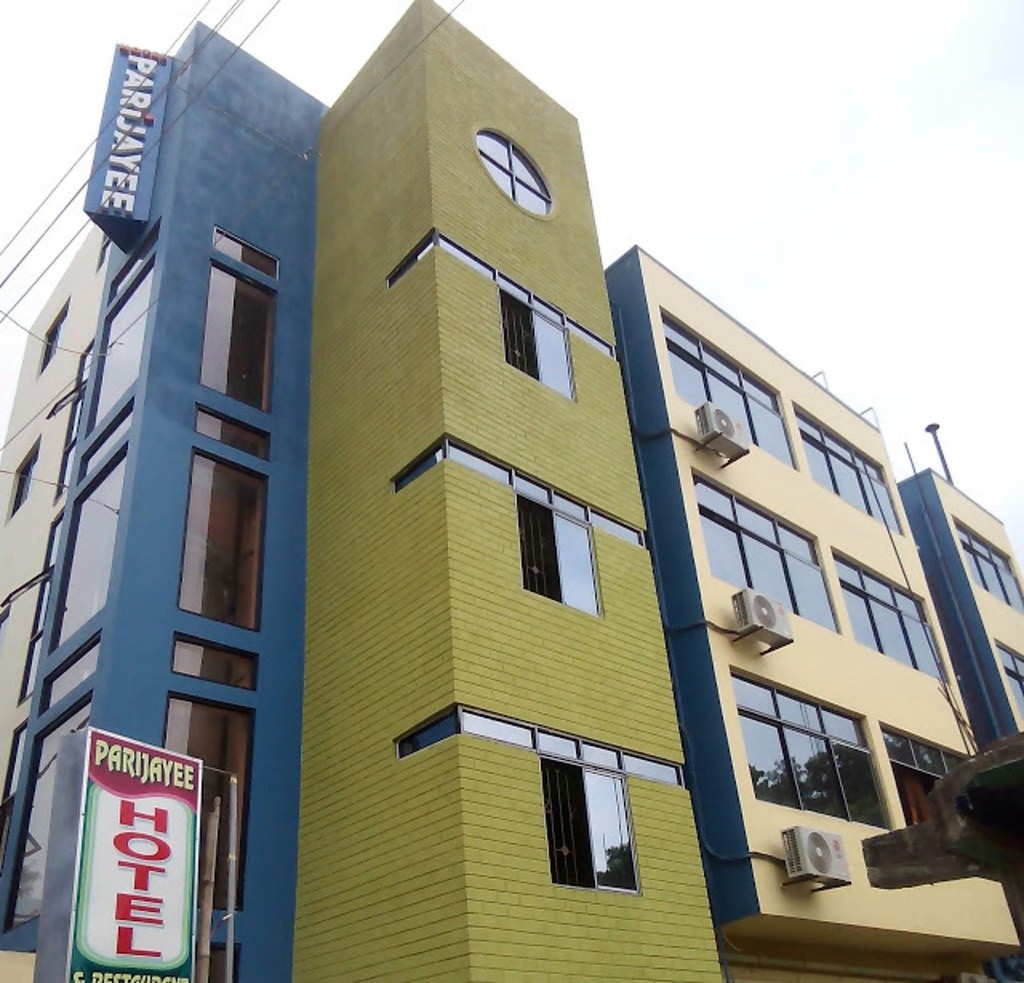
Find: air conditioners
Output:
[781,825,850,884]
[696,402,748,456]
[731,588,794,648]
